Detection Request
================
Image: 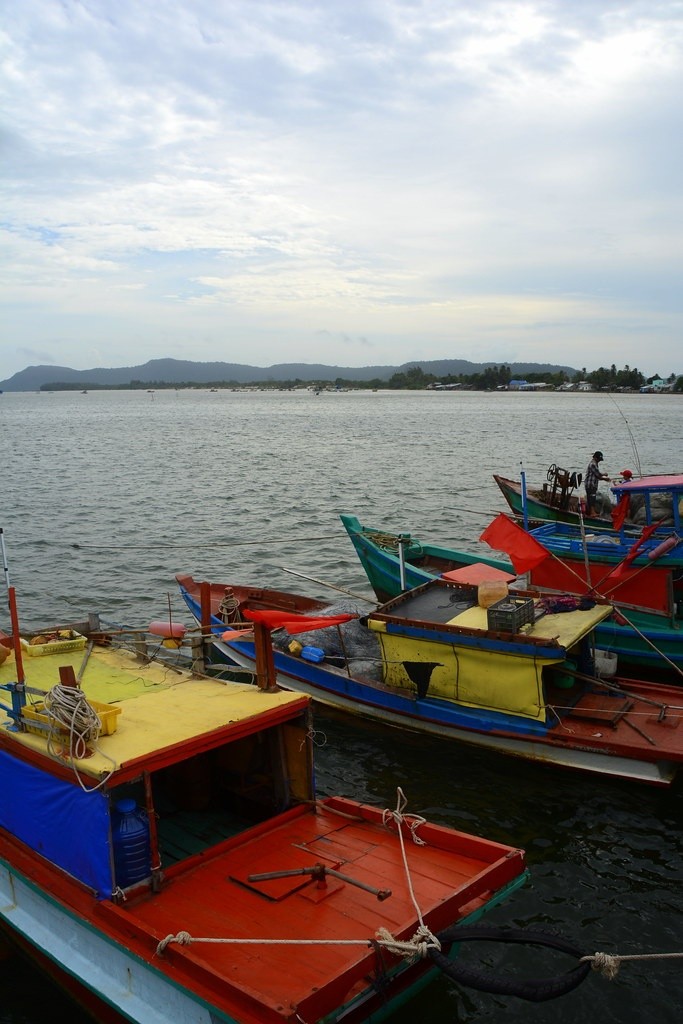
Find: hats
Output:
[594,451,603,461]
[620,470,632,478]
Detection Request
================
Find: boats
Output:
[0,526,535,1024]
[519,471,683,574]
[339,512,683,684]
[173,569,683,788]
[494,473,641,535]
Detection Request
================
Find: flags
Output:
[480,513,553,575]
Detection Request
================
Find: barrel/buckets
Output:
[301,645,325,663]
[585,534,594,543]
[478,581,508,607]
[579,502,586,511]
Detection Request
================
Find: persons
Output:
[613,470,634,496]
[584,451,612,519]
